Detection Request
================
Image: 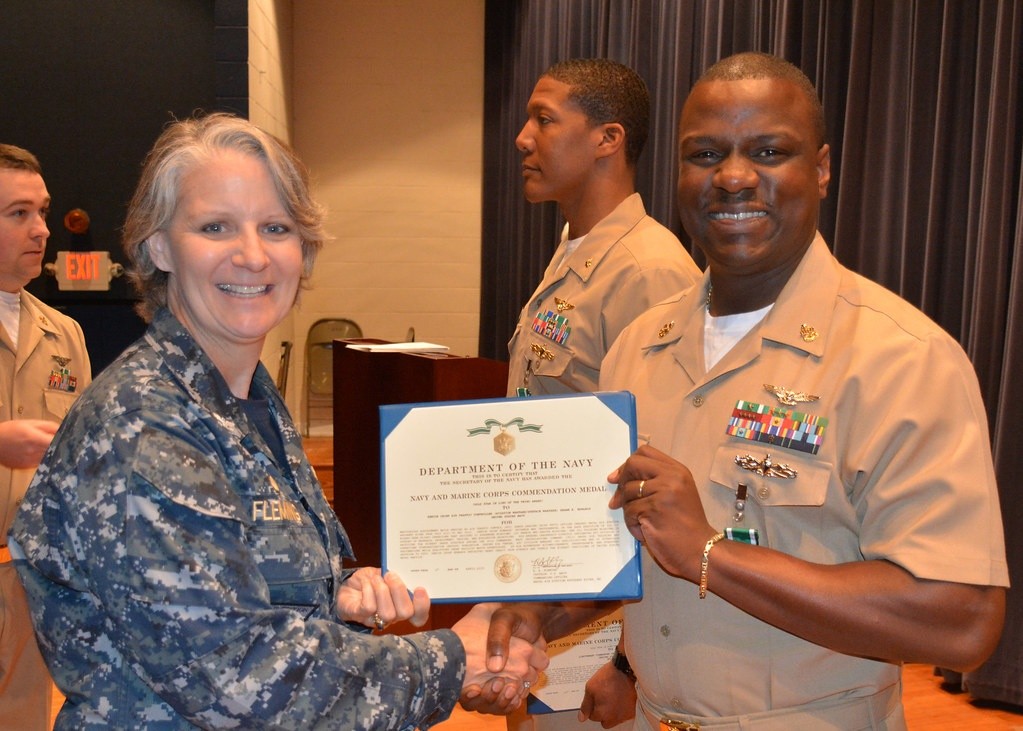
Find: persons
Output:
[458,51,1023,731]
[0,140,93,731]
[9,109,544,731]
[485,59,710,731]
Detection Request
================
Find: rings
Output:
[638,479,645,499]
[518,680,532,698]
[372,612,390,630]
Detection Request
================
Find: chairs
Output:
[305,318,416,439]
[277,341,293,401]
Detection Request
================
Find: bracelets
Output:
[612,644,638,684]
[697,531,726,600]
